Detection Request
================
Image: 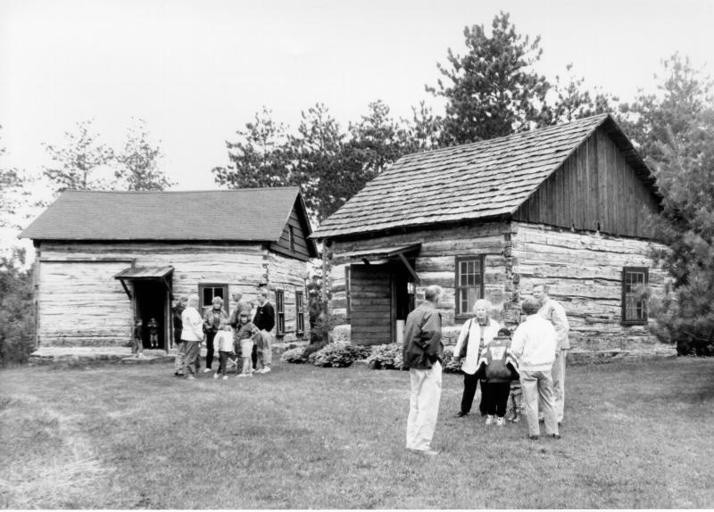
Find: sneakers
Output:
[455,410,565,439]
[403,447,440,457]
[174,366,271,380]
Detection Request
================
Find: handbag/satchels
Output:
[460,334,469,358]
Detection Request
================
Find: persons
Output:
[147,317,159,347]
[131,319,143,358]
[402,285,445,455]
[479,327,519,427]
[531,282,569,426]
[171,286,274,380]
[511,299,561,441]
[452,299,498,416]
